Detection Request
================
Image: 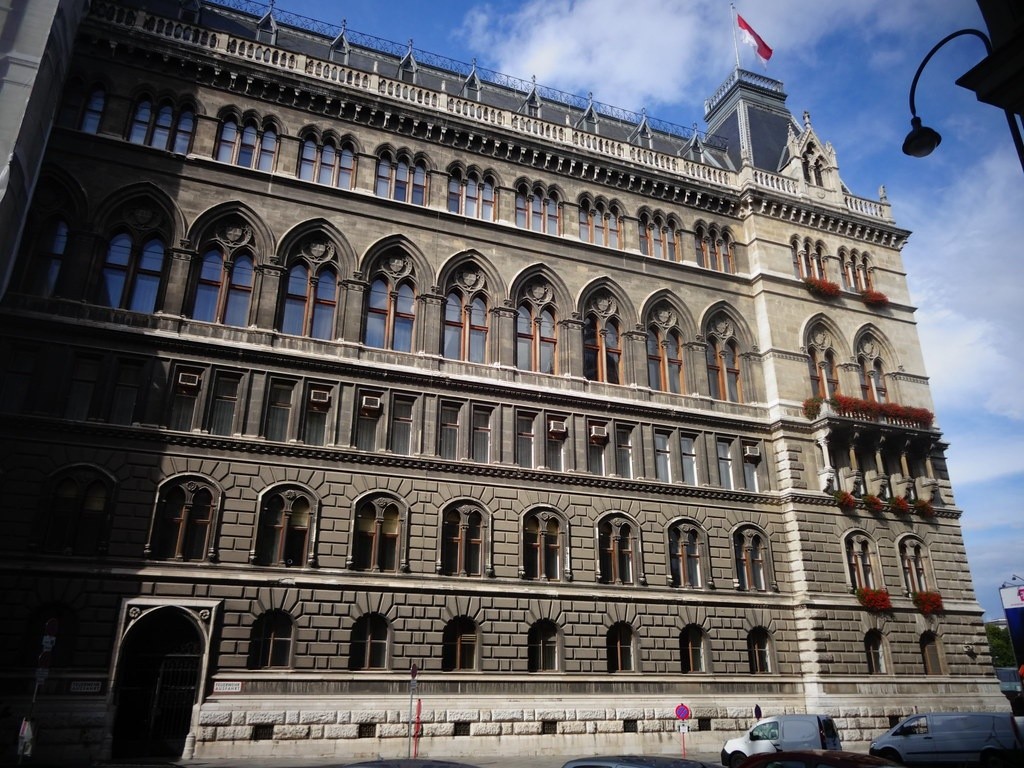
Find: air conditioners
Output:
[590,425,607,438]
[310,390,329,401]
[550,421,565,433]
[179,373,198,386]
[744,446,760,457]
[362,396,380,410]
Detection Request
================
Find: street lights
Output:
[902,28,1024,170]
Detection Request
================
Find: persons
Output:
[1017,589,1024,601]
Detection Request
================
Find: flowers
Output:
[913,498,936,518]
[863,493,883,511]
[833,488,856,510]
[854,588,894,612]
[803,394,936,428]
[802,274,841,298]
[912,590,944,613]
[889,495,907,513]
[860,288,889,306]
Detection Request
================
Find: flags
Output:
[738,14,773,71]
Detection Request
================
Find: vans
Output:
[721,713,843,768]
[868,711,1024,768]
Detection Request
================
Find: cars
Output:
[562,755,728,768]
[736,749,909,768]
[995,666,1023,707]
